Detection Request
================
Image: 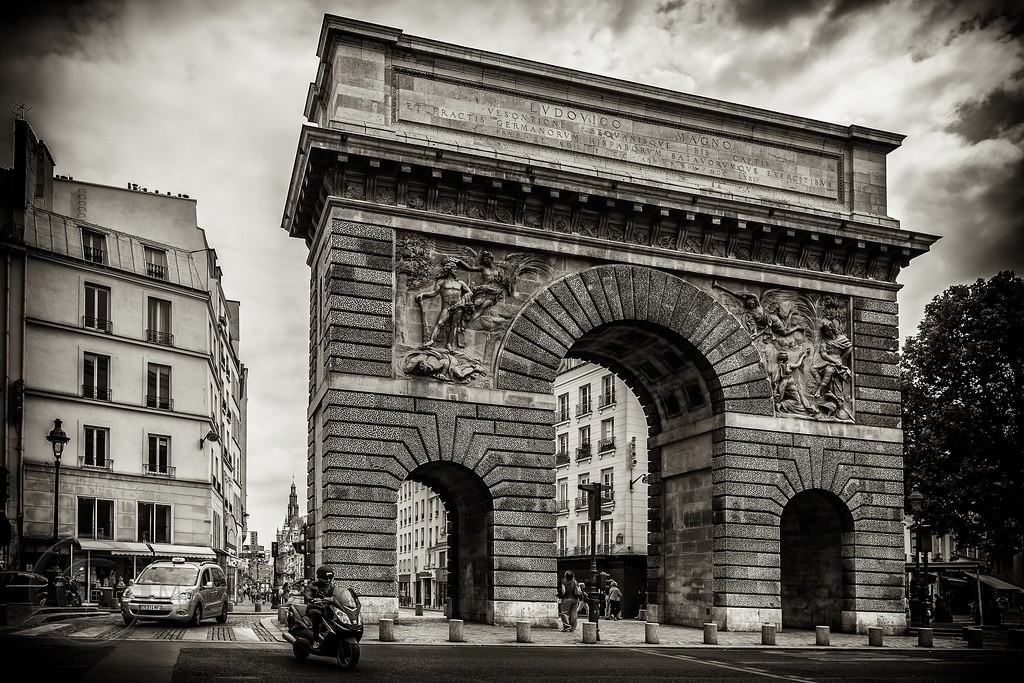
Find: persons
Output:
[603,578,623,621]
[413,262,474,353]
[455,249,505,321]
[302,564,341,648]
[575,582,590,620]
[239,583,262,603]
[561,570,577,631]
[770,347,811,411]
[738,291,804,337]
[811,320,853,397]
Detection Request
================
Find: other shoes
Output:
[313,640,320,649]
[617,617,622,620]
[611,615,617,621]
[563,626,572,632]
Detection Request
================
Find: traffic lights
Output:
[599,572,612,590]
[292,542,301,553]
[578,482,612,521]
[0,466,11,509]
[272,542,279,557]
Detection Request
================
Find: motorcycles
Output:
[282,585,363,669]
[54,567,85,606]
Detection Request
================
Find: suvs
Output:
[121,558,228,627]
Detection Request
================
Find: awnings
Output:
[78,540,219,559]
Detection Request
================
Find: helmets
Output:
[317,566,334,579]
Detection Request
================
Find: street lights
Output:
[45,416,69,598]
[908,483,929,627]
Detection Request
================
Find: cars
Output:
[0,570,48,606]
[991,597,1023,617]
